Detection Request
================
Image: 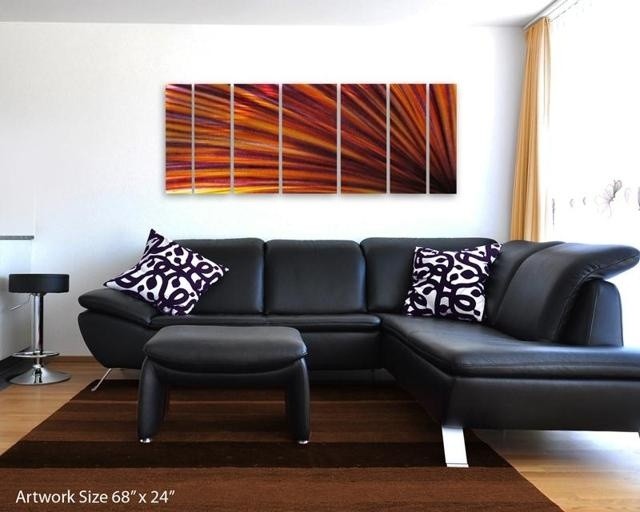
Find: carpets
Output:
[1,378,565,512]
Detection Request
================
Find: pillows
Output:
[103,227,230,317]
[402,241,502,322]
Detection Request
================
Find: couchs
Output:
[78,237,639,434]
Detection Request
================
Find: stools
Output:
[8,273,69,385]
[136,324,310,445]
